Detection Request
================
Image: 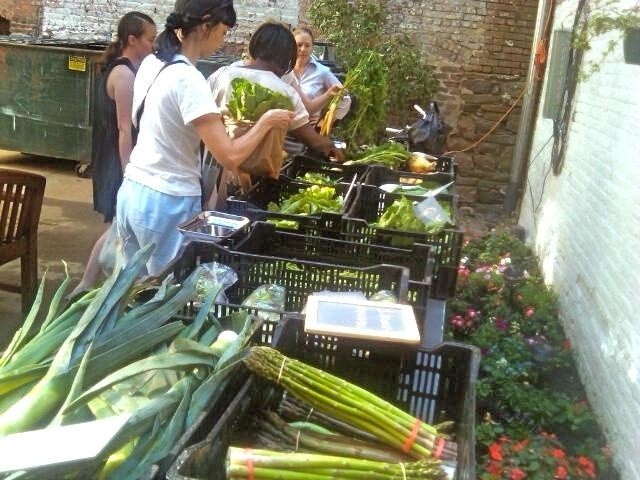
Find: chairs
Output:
[0,164,47,311]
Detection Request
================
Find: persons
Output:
[231,16,343,115]
[63,11,156,306]
[204,22,347,210]
[284,28,352,158]
[112,0,298,284]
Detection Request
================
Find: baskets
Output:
[125,145,483,480]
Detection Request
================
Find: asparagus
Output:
[225,344,458,480]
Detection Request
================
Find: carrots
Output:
[319,103,337,136]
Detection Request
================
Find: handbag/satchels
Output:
[407,100,454,155]
